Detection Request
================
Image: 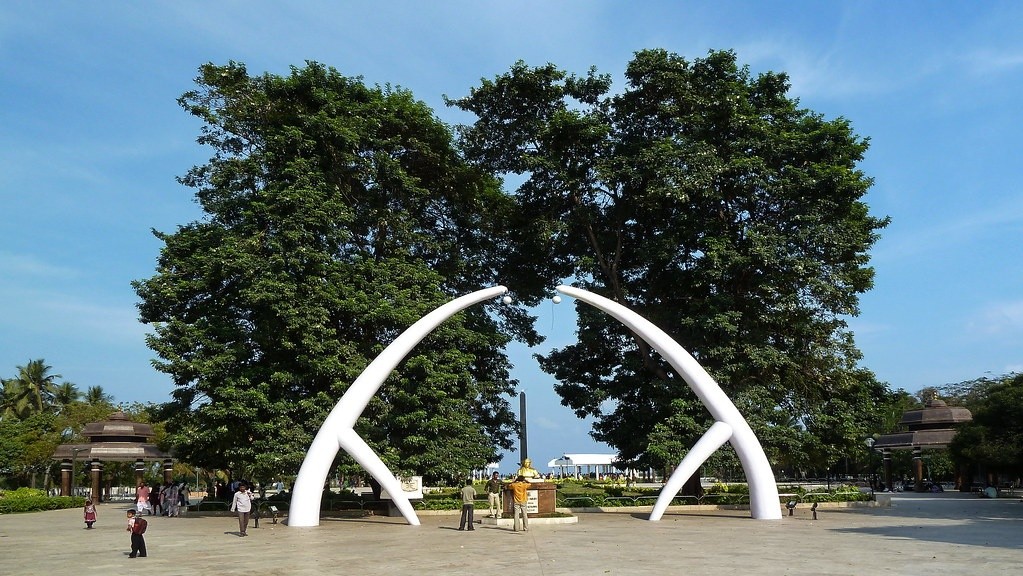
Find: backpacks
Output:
[131,518,148,535]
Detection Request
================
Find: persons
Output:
[870,471,1022,500]
[483,471,514,518]
[83,499,97,529]
[459,479,477,530]
[446,471,635,488]
[517,458,540,478]
[325,476,365,494]
[508,475,532,532]
[289,479,296,490]
[276,479,285,494]
[136,480,192,517]
[209,477,268,501]
[126,509,147,557]
[229,483,254,537]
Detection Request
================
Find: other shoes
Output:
[244,534,248,536]
[240,532,244,537]
